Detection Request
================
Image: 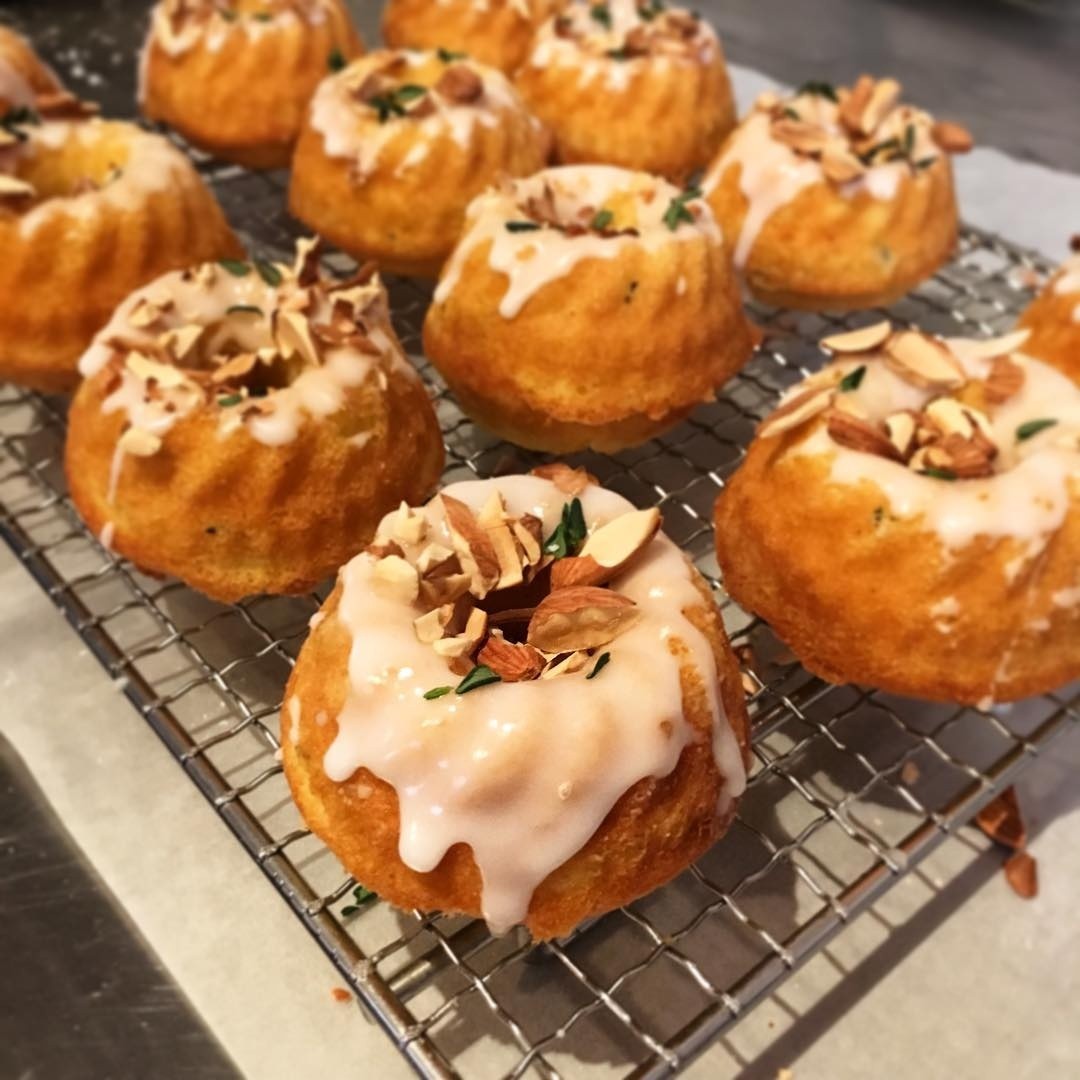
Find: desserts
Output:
[0,0,1080,932]
